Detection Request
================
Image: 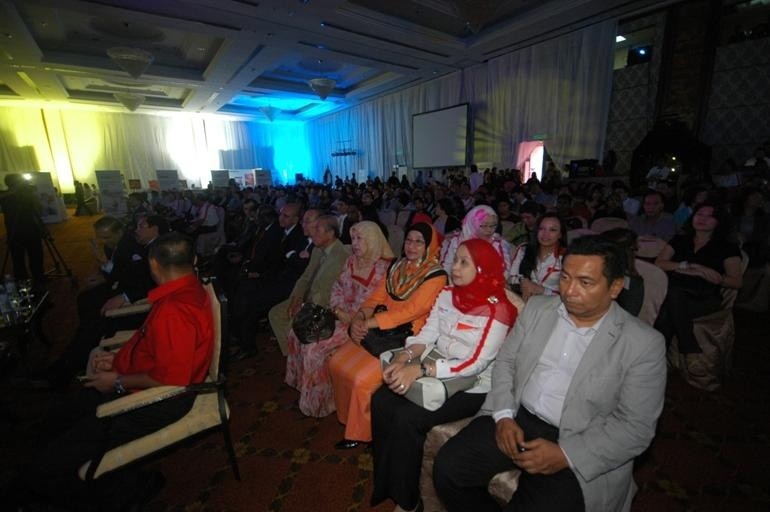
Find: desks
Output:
[0,288,53,362]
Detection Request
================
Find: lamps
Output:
[113,94,146,111]
[106,47,153,80]
[308,78,336,100]
[451,1,498,31]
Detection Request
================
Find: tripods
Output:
[1,228,69,278]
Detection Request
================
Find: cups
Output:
[3,278,36,326]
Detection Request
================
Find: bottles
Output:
[0,274,17,314]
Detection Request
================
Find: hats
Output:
[406,223,432,249]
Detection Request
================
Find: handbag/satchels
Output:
[290,303,335,343]
[380,345,481,413]
[359,302,413,358]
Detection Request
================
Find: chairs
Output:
[66,172,770,511]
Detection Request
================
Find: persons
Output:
[0,139,769,511]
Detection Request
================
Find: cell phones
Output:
[516,444,528,452]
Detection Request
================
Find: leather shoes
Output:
[371,486,423,512]
[335,438,357,449]
[685,353,705,376]
[230,349,257,361]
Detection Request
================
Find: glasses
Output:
[404,238,425,246]
[479,224,497,229]
